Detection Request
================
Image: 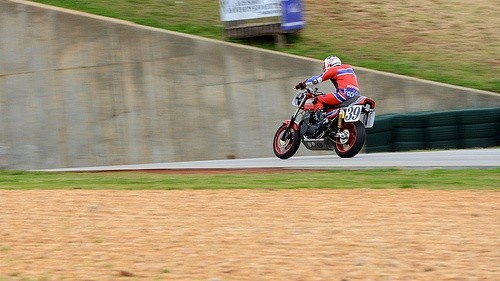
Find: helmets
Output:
[323,55,341,71]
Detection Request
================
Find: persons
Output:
[294,55,360,127]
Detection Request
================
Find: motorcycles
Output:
[272,86,376,160]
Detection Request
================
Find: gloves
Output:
[294,80,306,89]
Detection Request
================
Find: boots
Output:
[314,110,328,130]
